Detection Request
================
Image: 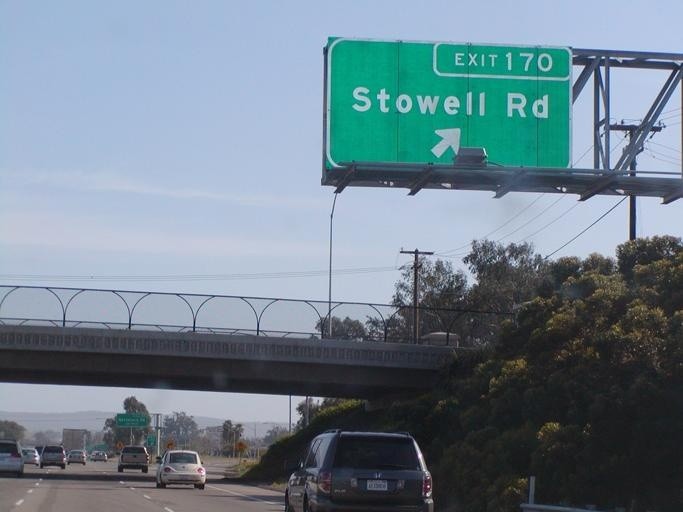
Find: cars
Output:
[285,428,434,512]
[41,446,66,468]
[0,438,23,476]
[91,451,107,461]
[156,450,206,490]
[66,450,86,466]
[21,448,40,467]
[118,446,150,472]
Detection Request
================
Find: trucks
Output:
[63,430,86,452]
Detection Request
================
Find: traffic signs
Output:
[325,37,572,173]
[147,435,157,447]
[116,413,149,427]
